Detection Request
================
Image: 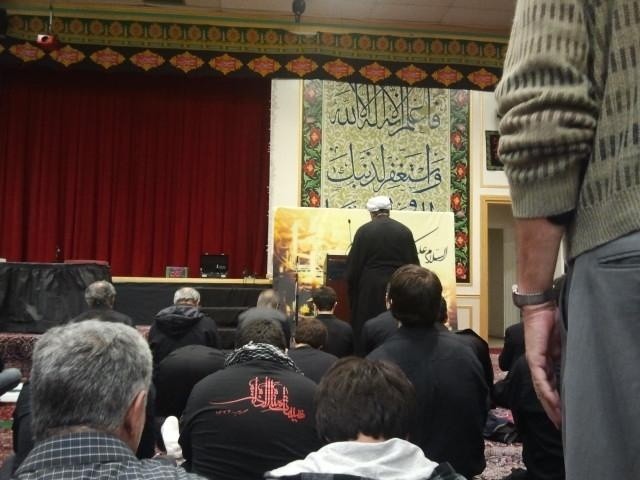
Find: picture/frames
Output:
[112,276,274,329]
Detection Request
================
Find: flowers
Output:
[348,218,352,245]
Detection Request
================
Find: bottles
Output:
[367,196,391,212]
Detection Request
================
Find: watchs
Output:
[512,282,557,307]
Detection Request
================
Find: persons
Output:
[12,377,35,473]
[496,0,640,479]
[355,284,399,358]
[364,264,491,479]
[148,287,222,366]
[434,295,496,410]
[263,354,461,477]
[74,280,137,327]
[343,195,419,351]
[177,316,327,479]
[493,325,567,478]
[312,286,355,357]
[12,316,201,478]
[498,321,526,371]
[288,315,339,385]
[235,289,291,350]
[156,344,227,472]
